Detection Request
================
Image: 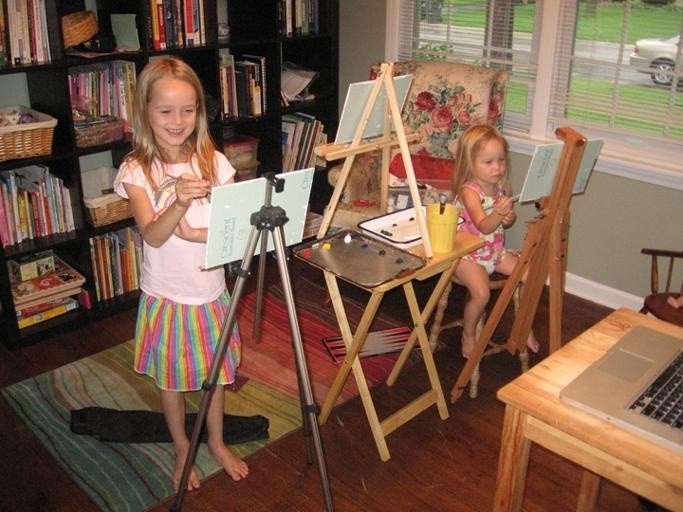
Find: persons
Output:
[443,124,542,362]
[111,56,251,491]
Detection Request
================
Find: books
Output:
[68,60,138,134]
[2,2,51,65]
[1,165,75,249]
[10,255,87,326]
[221,130,261,184]
[282,112,324,175]
[88,225,146,302]
[96,2,206,52]
[278,2,319,36]
[219,54,267,119]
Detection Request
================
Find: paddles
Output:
[2,272,449,512]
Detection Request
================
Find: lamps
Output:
[323,61,512,308]
[387,186,529,399]
[638,248,683,330]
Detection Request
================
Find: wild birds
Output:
[559,327,682,450]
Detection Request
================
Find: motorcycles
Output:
[176,211,336,508]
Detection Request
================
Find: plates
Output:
[1,1,338,351]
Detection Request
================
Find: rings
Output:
[165,171,211,195]
[481,193,520,211]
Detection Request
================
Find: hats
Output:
[626,32,683,90]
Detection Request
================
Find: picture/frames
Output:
[493,307,683,511]
[314,219,485,461]
